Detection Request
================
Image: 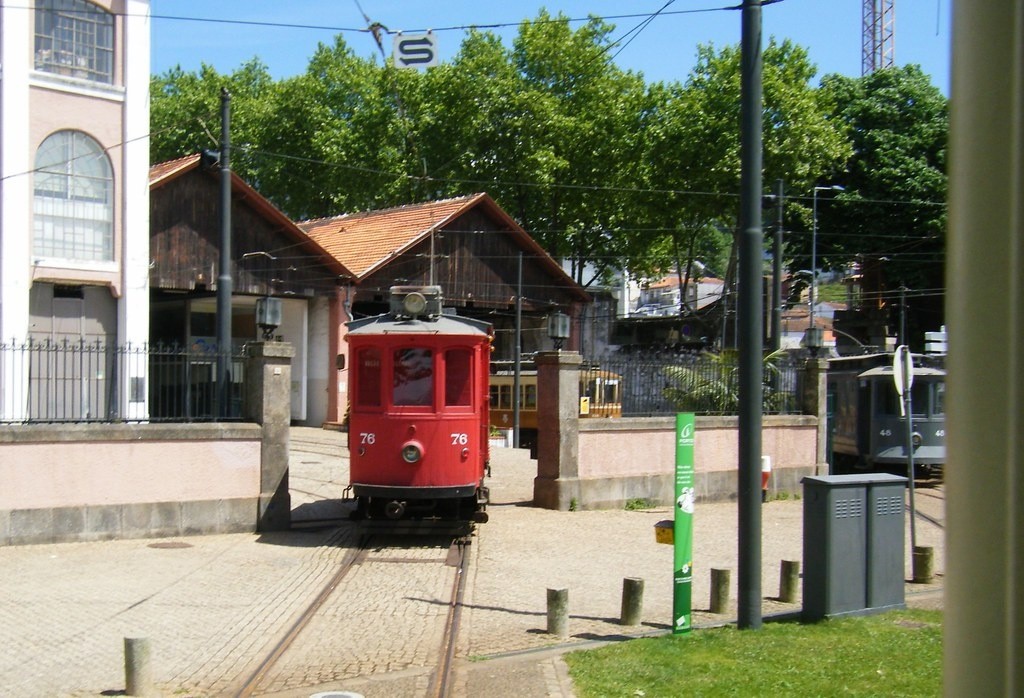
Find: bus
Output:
[333,271,492,540]
[825,342,948,480]
[487,348,622,445]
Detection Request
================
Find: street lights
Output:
[808,186,848,327]
